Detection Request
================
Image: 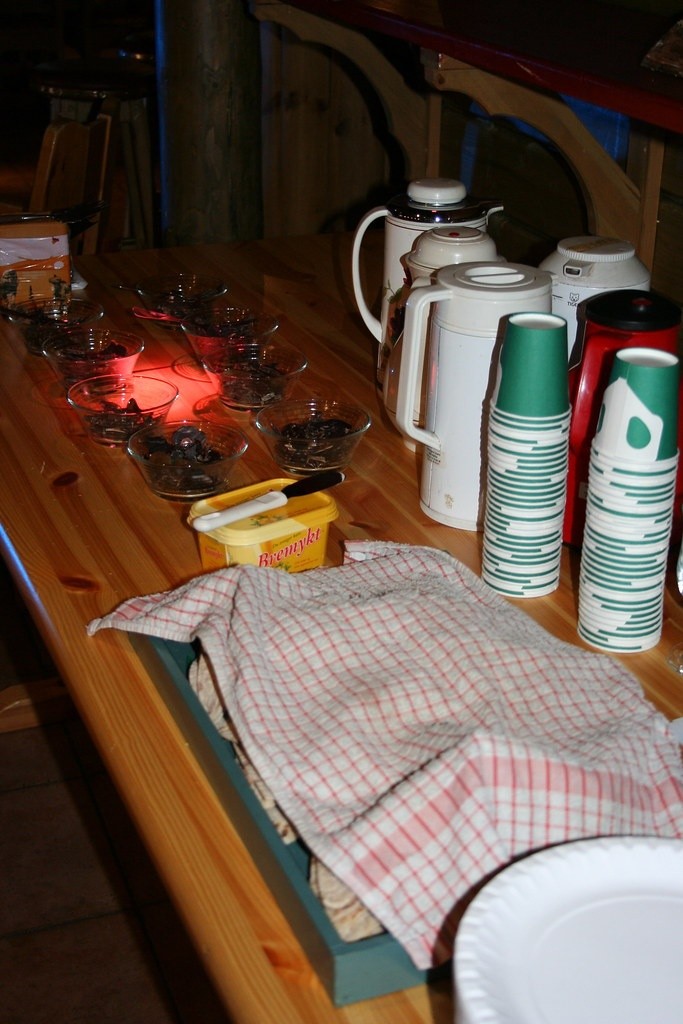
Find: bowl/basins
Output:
[7,299,104,355]
[256,397,372,480]
[41,329,145,389]
[127,420,250,502]
[182,305,281,365]
[137,274,229,330]
[201,343,308,412]
[66,374,179,446]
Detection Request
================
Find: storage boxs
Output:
[187,479,340,574]
[0,212,71,302]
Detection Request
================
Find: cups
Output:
[481,312,572,597]
[578,346,681,654]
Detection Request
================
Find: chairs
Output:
[0,113,114,255]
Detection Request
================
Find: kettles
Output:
[562,290,683,546]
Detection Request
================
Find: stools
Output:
[36,69,154,249]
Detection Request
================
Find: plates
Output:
[454,835,683,1024]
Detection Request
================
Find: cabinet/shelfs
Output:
[250,0,683,272]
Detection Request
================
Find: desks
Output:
[0,229,683,1024]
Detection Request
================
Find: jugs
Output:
[383,226,507,453]
[395,262,562,531]
[352,178,504,402]
